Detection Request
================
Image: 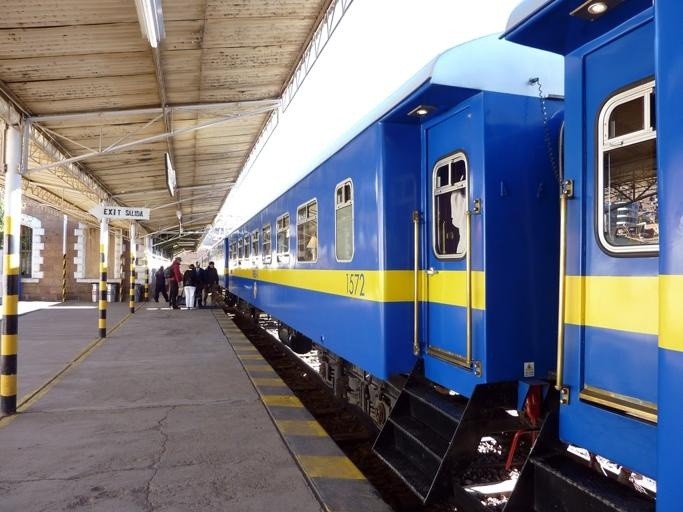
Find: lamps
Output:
[134,0,166,49]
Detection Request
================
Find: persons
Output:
[149,257,220,309]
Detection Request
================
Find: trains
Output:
[198,1,683,512]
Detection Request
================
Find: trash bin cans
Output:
[91,283,117,302]
[134,284,144,302]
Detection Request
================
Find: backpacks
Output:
[164,267,174,278]
